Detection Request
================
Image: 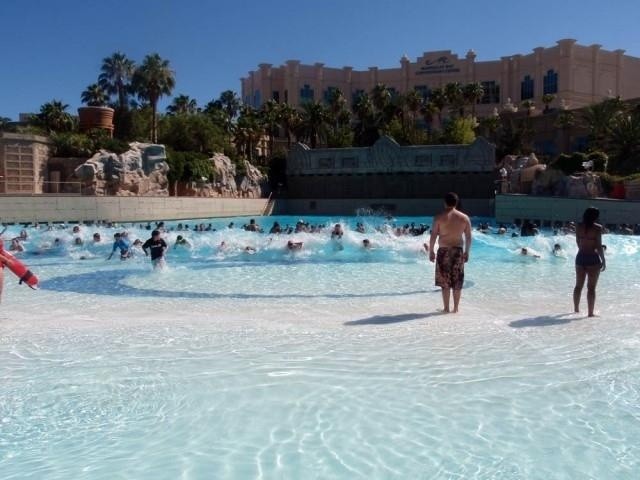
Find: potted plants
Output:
[420,103,442,132]
[542,93,557,114]
[623,172,640,200]
[520,99,536,117]
[78,85,115,131]
[608,175,627,199]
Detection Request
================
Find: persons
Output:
[220,241,226,248]
[396,222,427,236]
[246,246,255,250]
[477,219,577,255]
[573,206,607,318]
[143,231,167,263]
[605,223,640,235]
[106,232,130,260]
[11,221,193,252]
[271,220,370,250]
[228,220,263,232]
[192,223,215,231]
[429,191,472,313]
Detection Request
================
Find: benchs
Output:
[500,109,527,121]
